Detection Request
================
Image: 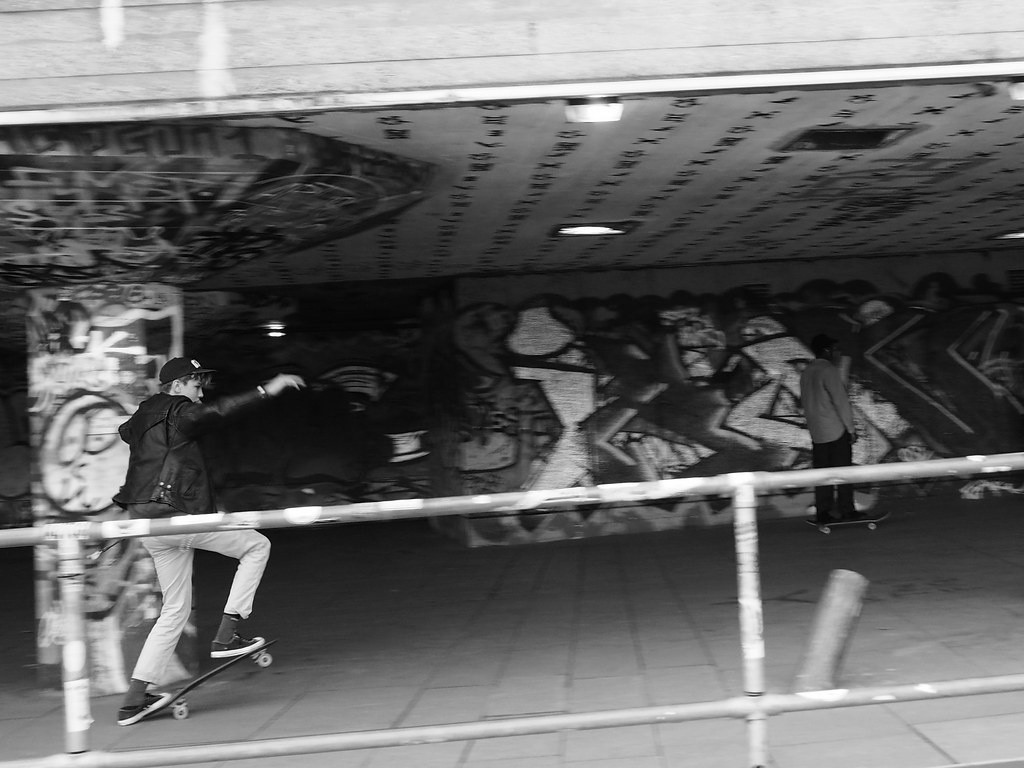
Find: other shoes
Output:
[840,511,868,521]
[818,511,834,523]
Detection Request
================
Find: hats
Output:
[158,356,216,384]
[811,333,839,353]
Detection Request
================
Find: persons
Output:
[800,333,864,525]
[110,358,307,726]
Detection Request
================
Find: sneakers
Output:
[210,633,265,659]
[117,692,172,726]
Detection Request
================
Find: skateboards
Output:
[136,634,280,722]
[806,508,892,534]
